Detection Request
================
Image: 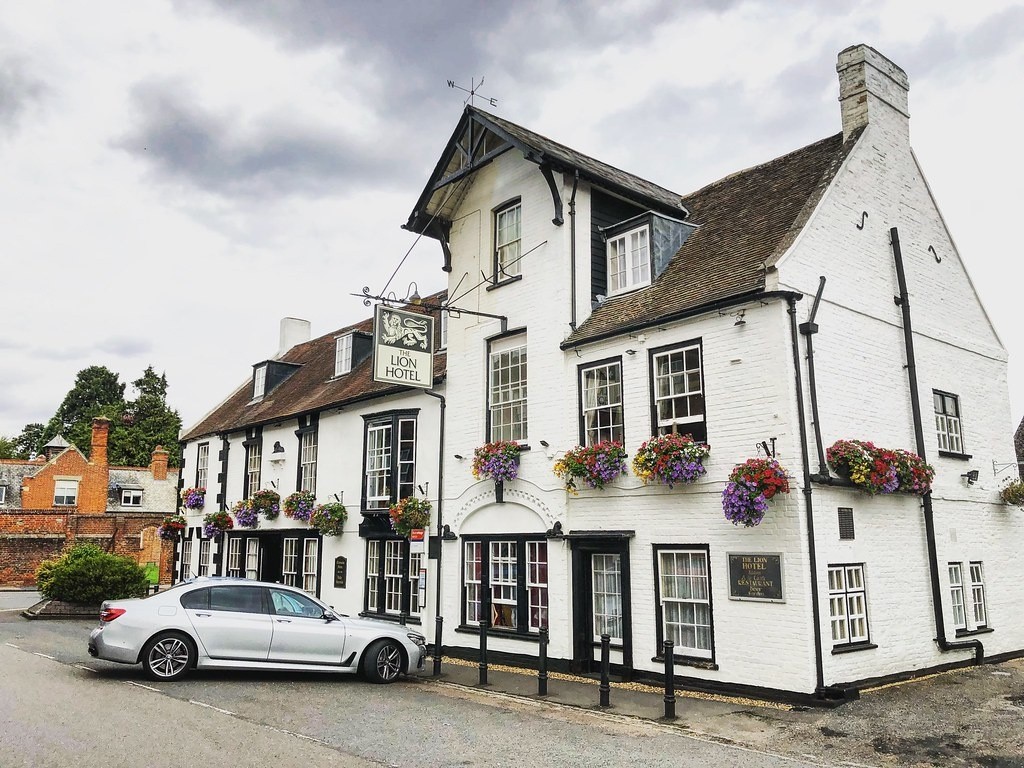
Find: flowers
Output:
[182,487,206,511]
[157,515,187,541]
[232,498,260,529]
[826,440,936,499]
[282,490,317,523]
[309,502,348,536]
[998,474,1024,513]
[388,496,433,543]
[721,455,789,529]
[631,433,710,491]
[550,439,630,496]
[472,441,521,485]
[250,489,281,520]
[203,510,234,539]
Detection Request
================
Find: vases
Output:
[834,463,852,478]
[1004,490,1017,505]
[511,454,520,467]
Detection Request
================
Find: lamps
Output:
[540,441,549,447]
[626,350,637,355]
[961,470,978,485]
[734,321,746,326]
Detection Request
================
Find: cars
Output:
[87,575,427,686]
